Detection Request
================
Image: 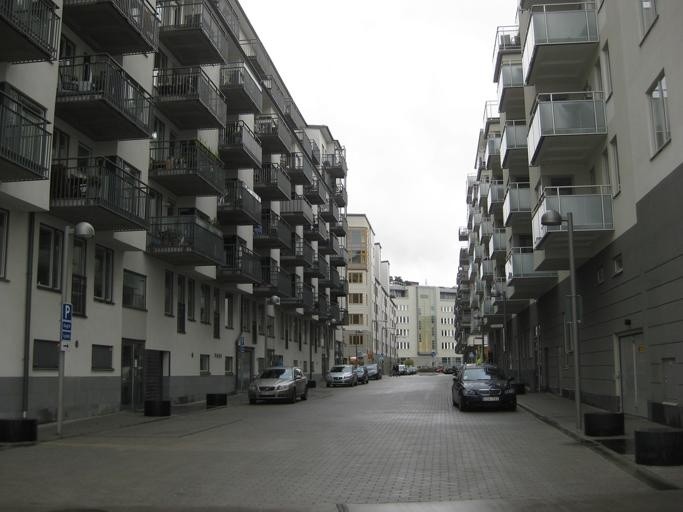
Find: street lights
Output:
[57,221,94,436]
[542,210,583,429]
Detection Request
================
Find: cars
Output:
[248,366,309,404]
[325,363,382,387]
[452,363,517,410]
